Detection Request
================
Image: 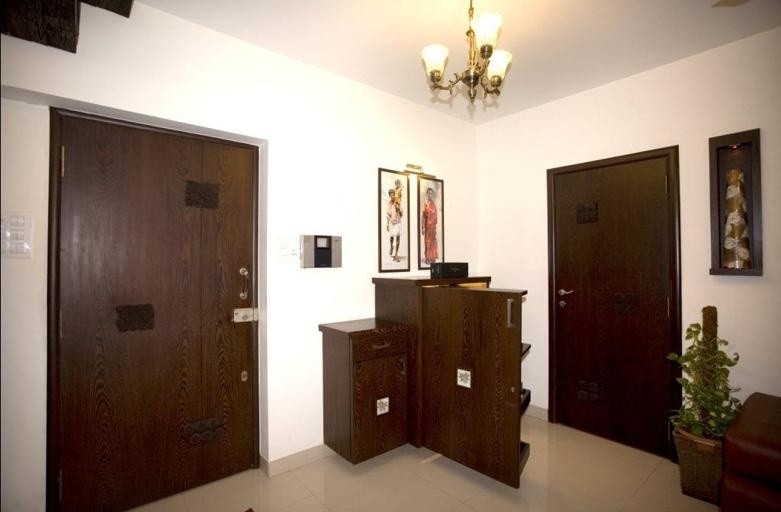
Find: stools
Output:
[720,391,781,511]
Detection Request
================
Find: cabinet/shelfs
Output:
[319,318,411,465]
[419,287,531,489]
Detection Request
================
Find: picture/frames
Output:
[417,176,445,270]
[378,168,410,273]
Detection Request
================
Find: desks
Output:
[372,276,491,448]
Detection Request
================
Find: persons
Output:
[422,189,439,264]
[387,178,403,262]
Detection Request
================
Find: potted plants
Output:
[667,305,743,506]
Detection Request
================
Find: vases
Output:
[722,168,753,271]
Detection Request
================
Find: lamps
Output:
[420,0,513,105]
